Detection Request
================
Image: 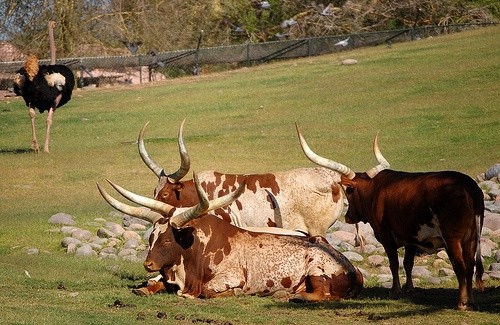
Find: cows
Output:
[96,171,363,304]
[294,121,485,311]
[106,179,312,285]
[137,119,366,251]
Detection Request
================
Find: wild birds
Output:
[150,51,166,69]
[260,0,271,8]
[284,18,298,28]
[321,3,334,17]
[334,36,350,48]
[118,39,143,54]
